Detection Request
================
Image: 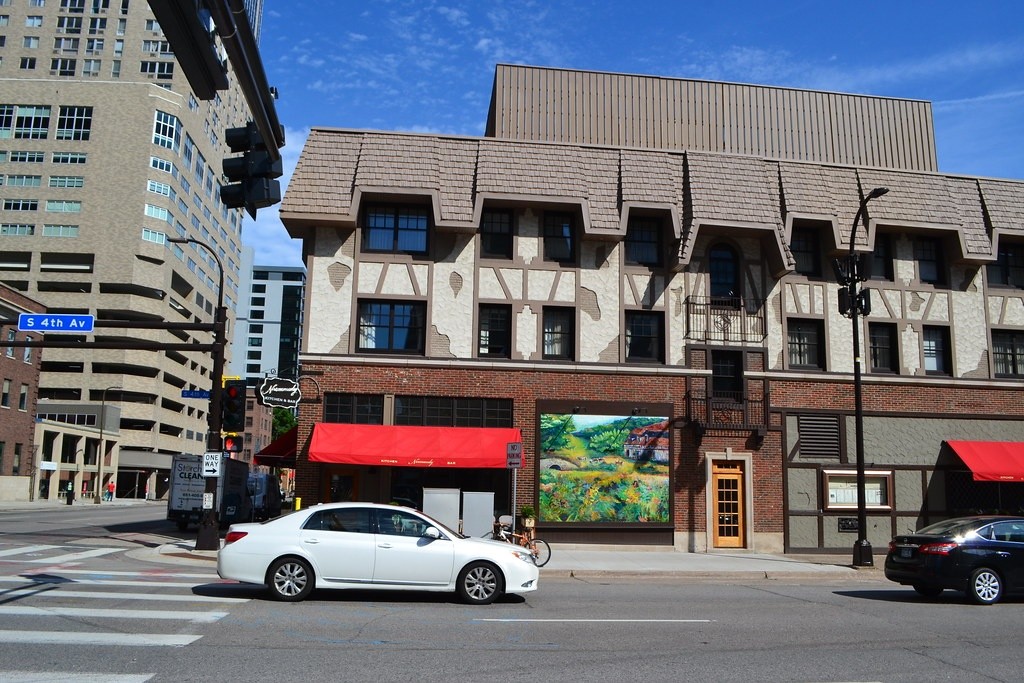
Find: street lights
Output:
[96,385,124,503]
[167,235,225,461]
[848,186,892,567]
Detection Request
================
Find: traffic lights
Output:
[216,121,283,223]
[223,435,243,451]
[221,380,249,434]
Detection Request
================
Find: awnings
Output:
[944,441,1024,483]
[309,423,526,469]
[255,426,298,467]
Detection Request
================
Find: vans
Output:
[246,472,282,522]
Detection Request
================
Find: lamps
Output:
[573,407,586,414]
[632,408,647,414]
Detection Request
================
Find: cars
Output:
[215,501,539,607]
[280,489,293,507]
[884,514,1024,604]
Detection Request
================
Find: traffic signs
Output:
[201,453,223,480]
[506,443,522,469]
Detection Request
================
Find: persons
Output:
[107,482,116,501]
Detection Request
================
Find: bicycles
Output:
[493,519,552,568]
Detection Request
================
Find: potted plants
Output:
[520,497,540,527]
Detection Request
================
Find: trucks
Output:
[167,452,258,530]
